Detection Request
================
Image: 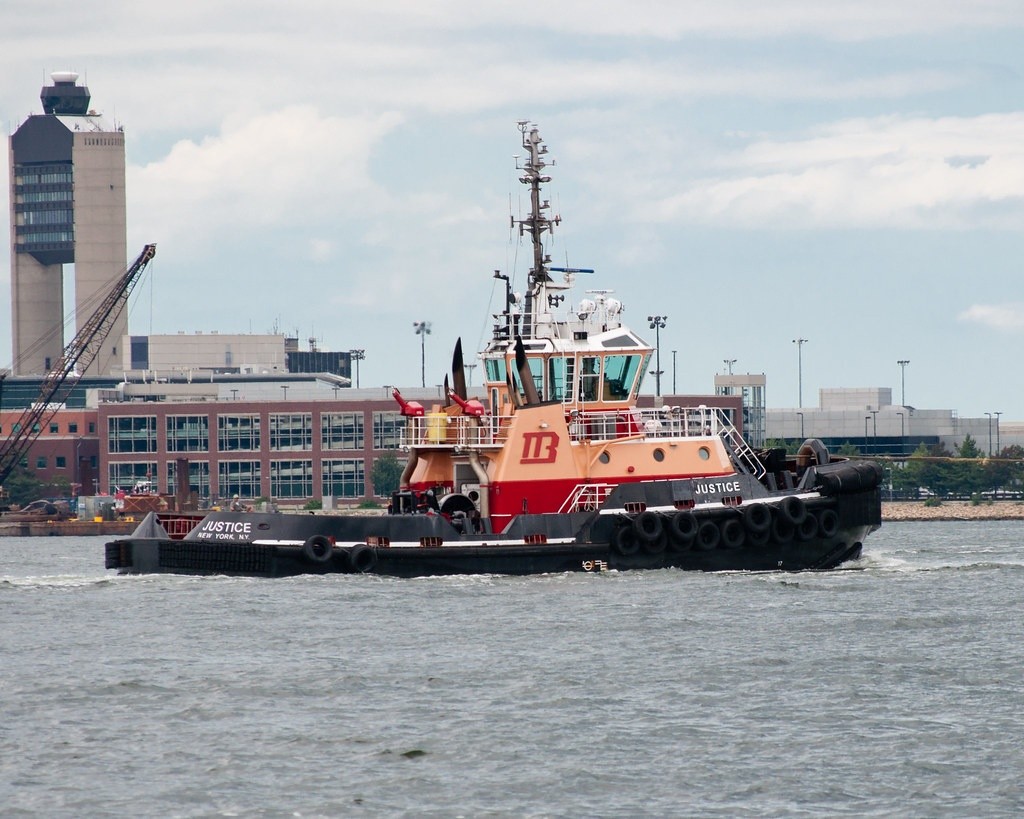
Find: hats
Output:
[233,494,239,498]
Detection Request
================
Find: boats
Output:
[104,118,882,579]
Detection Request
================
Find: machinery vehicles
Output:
[0,244,157,515]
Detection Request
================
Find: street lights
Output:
[647,314,668,395]
[865,416,872,456]
[896,360,911,435]
[796,412,804,438]
[994,412,1003,455]
[868,410,880,454]
[792,339,809,437]
[984,412,992,459]
[415,321,430,385]
[897,412,905,434]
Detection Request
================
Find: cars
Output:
[17,498,77,517]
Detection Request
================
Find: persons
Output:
[246,506,255,513]
[230,494,247,513]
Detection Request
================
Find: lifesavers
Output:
[743,503,772,530]
[633,511,663,543]
[796,513,818,540]
[611,526,642,556]
[669,536,693,551]
[350,544,378,573]
[672,511,699,540]
[780,496,808,525]
[305,535,333,562]
[771,518,795,543]
[696,520,721,551]
[643,529,667,554]
[722,518,746,547]
[818,509,840,538]
[748,531,771,545]
